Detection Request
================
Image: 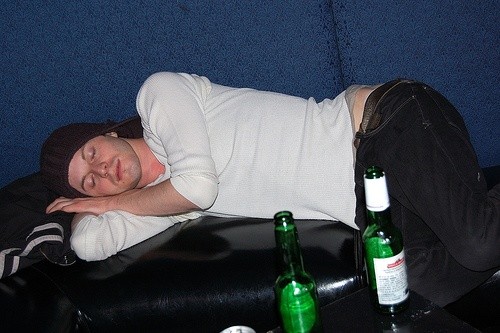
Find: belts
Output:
[352,78,414,163]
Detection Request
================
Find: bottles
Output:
[272,211,324,333]
[362,166,410,315]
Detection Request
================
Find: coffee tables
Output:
[267,281,481,333]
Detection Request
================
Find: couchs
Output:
[0,0,500,332]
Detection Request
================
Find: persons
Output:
[40,69,500,307]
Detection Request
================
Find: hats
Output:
[38,114,141,200]
